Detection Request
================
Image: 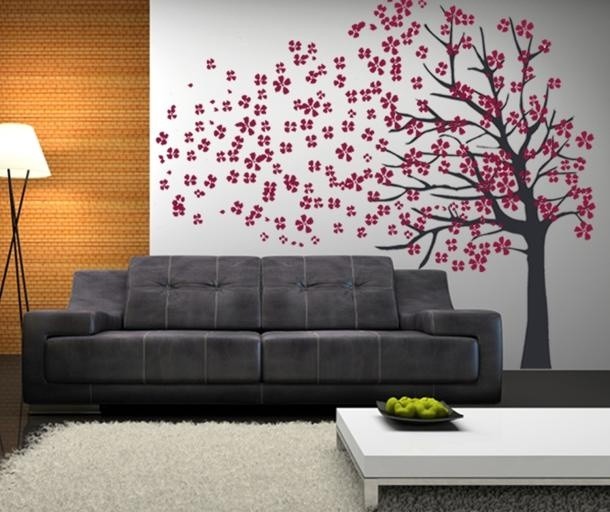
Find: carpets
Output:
[1,421,609,512]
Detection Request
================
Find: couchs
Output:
[24,256,504,419]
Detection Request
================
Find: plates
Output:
[375,399,462,424]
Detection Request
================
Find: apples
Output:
[385,396,449,419]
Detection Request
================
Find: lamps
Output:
[0,122,50,323]
